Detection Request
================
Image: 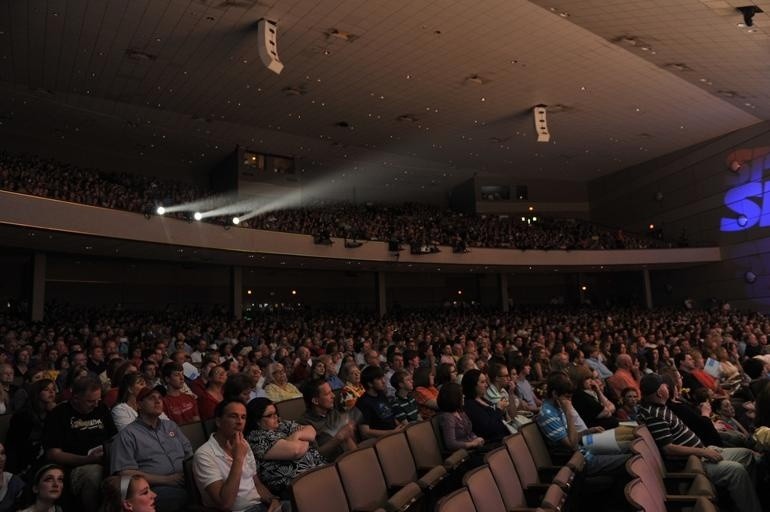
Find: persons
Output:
[0,156,673,250]
[436,309,770,512]
[0,309,435,512]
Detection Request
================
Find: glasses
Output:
[211,370,228,375]
[451,369,456,374]
[259,412,279,419]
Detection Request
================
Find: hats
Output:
[137,384,167,402]
[640,373,675,396]
